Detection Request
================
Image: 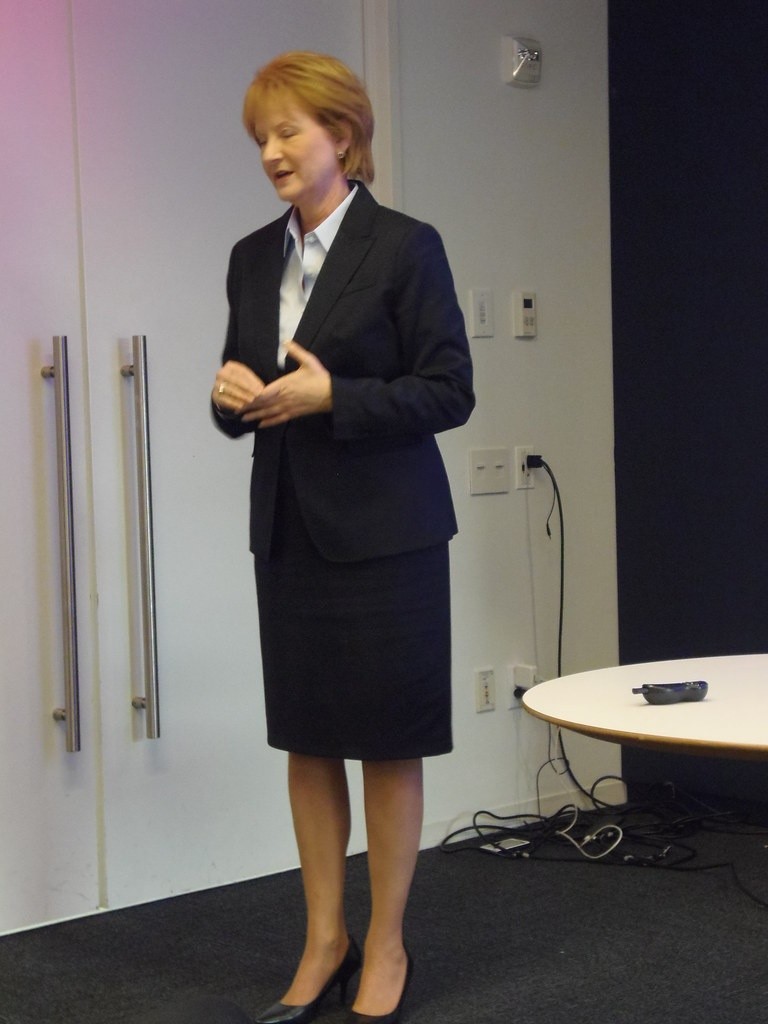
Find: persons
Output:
[210,50,478,1024]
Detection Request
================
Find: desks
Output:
[523,654,768,761]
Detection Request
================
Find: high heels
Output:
[348,945,410,1024]
[255,936,364,1024]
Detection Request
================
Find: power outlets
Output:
[514,445,536,490]
[474,668,495,713]
[508,664,525,709]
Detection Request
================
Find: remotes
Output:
[632,681,708,704]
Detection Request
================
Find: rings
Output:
[217,381,230,396]
